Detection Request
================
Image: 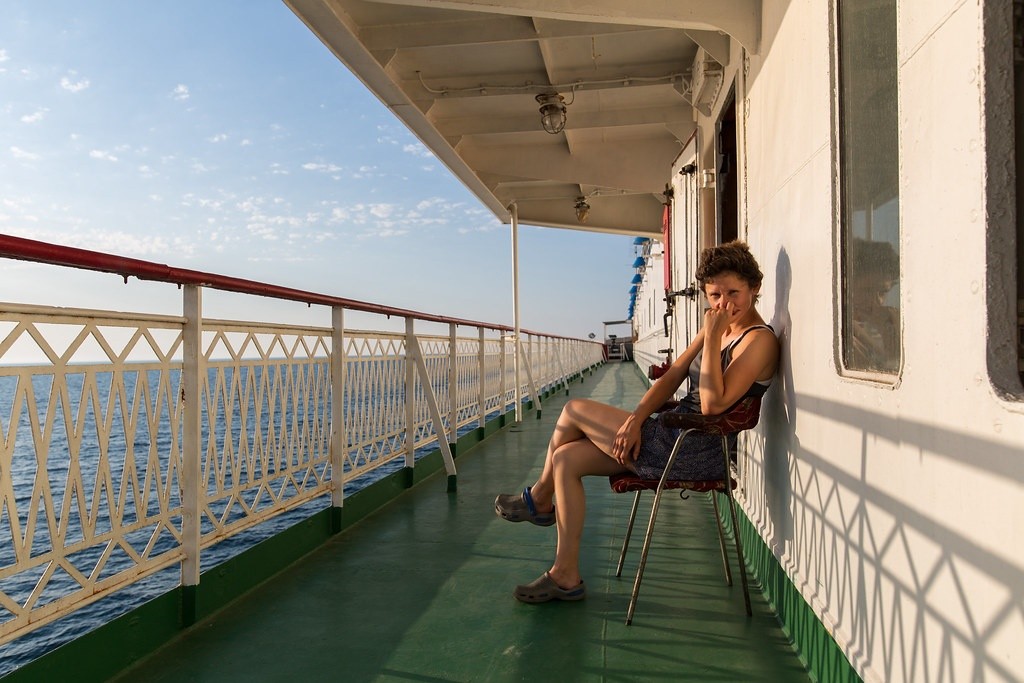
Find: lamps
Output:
[575,197,590,222]
[536,90,566,134]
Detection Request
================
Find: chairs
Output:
[610,396,764,626]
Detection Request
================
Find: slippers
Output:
[512,570,586,604]
[494,486,556,527]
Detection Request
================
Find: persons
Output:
[495,240,779,603]
